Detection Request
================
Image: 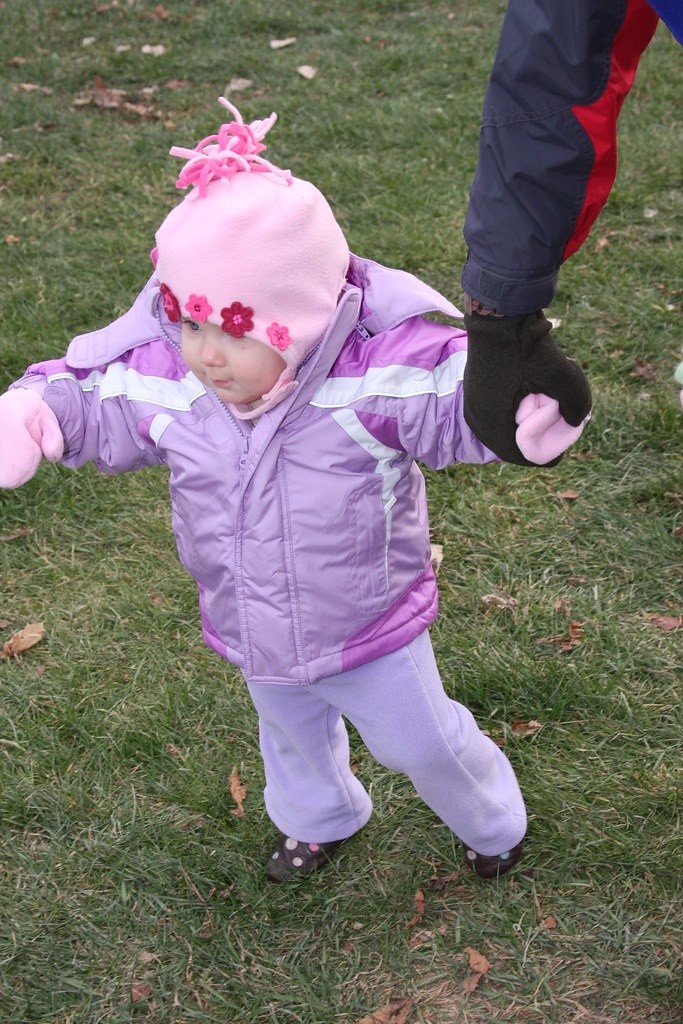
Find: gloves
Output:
[1,389,64,491]
[514,394,587,466]
[461,309,593,468]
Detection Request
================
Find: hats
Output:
[158,95,351,405]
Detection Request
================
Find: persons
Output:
[0,99,590,887]
[458,1,683,471]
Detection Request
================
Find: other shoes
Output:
[265,835,337,884]
[462,841,523,878]
